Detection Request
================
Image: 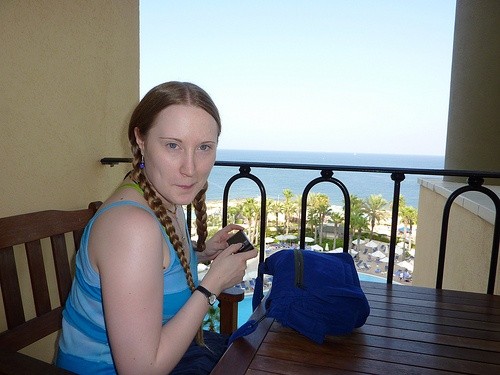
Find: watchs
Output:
[195,285,216,305]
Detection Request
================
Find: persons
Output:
[57,81,258,375]
[291,240,294,246]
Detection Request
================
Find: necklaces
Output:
[129,174,185,241]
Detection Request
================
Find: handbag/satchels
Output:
[265,249,370,342]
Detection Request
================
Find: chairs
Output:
[0,201,244,375]
[356,259,371,270]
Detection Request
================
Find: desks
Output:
[209,281,500,375]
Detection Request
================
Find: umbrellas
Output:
[396,242,415,270]
[352,239,365,245]
[365,241,377,247]
[371,250,389,262]
[305,237,314,242]
[266,234,297,245]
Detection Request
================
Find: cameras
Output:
[226,230,255,256]
